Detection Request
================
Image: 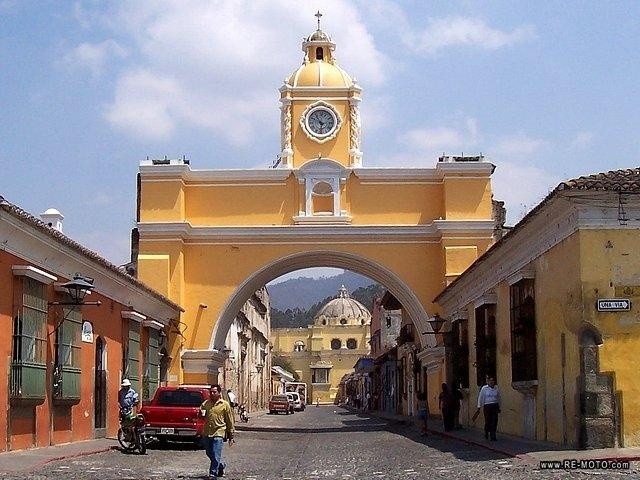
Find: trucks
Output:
[284,381,307,411]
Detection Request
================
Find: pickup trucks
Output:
[141,385,232,448]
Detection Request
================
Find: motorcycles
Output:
[118,392,150,454]
[235,401,251,422]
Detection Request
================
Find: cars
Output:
[268,392,304,415]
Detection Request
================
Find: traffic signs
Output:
[598,298,629,311]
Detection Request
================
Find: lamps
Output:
[248,364,264,380]
[417,311,452,338]
[45,271,102,308]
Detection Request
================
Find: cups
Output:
[201,409,206,417]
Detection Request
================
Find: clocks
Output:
[299,100,344,146]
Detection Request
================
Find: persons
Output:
[118,378,139,442]
[452,380,463,425]
[366,391,371,410]
[439,383,457,432]
[198,384,236,480]
[356,392,360,409]
[416,393,429,437]
[477,376,502,441]
[316,396,321,408]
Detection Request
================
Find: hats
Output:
[120,379,131,387]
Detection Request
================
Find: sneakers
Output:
[218,463,226,475]
[209,475,217,480]
